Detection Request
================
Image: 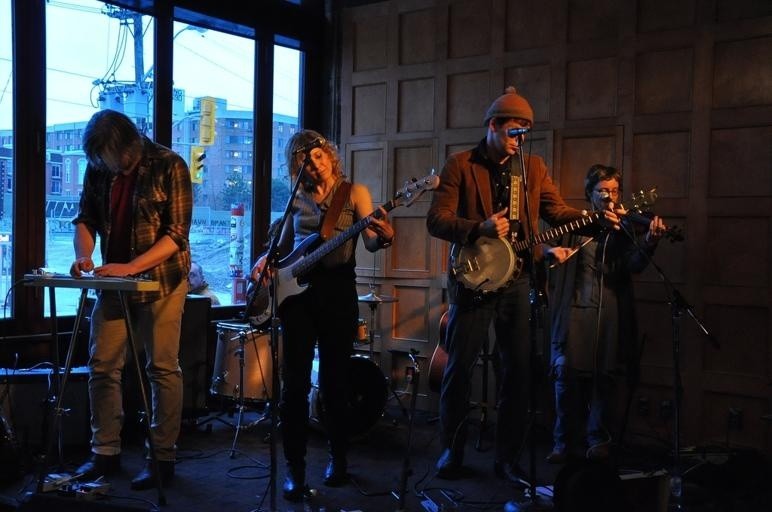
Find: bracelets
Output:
[374,235,397,250]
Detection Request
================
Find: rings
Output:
[655,226,661,235]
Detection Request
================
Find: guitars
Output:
[240,167,443,327]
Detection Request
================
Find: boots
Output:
[282,461,305,495]
[321,446,346,486]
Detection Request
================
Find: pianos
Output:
[22,264,163,296]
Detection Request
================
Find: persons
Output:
[539,163,666,465]
[183,260,223,307]
[247,128,399,498]
[425,85,628,484]
[69,109,194,490]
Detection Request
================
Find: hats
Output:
[483,86,534,130]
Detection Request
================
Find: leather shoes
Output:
[130,459,174,490]
[494,459,532,489]
[434,446,464,480]
[73,452,121,481]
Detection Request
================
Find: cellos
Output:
[427,308,450,392]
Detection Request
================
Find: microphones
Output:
[292,136,326,156]
[599,191,617,207]
[507,127,532,137]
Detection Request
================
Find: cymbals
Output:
[352,285,400,312]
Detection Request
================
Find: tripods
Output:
[182,336,281,457]
[423,331,546,451]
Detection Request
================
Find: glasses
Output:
[591,187,618,196]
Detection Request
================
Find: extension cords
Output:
[78,482,112,502]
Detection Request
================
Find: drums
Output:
[204,315,284,404]
[359,319,367,339]
